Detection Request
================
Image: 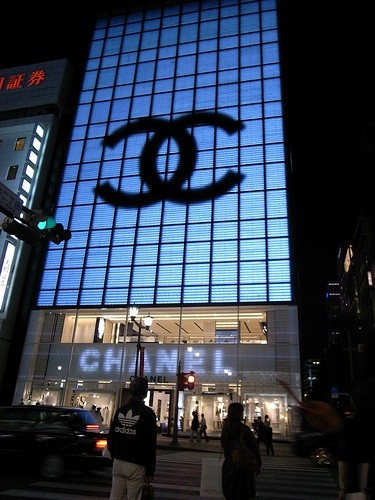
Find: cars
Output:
[1,405,108,480]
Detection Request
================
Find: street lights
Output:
[128,302,153,378]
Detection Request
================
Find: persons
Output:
[220,402,261,500]
[199,414,209,442]
[92,405,101,418]
[75,397,87,409]
[190,412,202,443]
[257,416,275,456]
[294,400,375,465]
[216,400,225,428]
[105,377,157,500]
[193,402,199,412]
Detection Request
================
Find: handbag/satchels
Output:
[142,485,154,500]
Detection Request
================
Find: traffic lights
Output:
[177,371,196,390]
[29,211,72,245]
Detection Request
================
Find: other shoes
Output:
[189,439,193,442]
[196,439,201,442]
[205,439,209,442]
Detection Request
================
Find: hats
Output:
[130,376,148,393]
